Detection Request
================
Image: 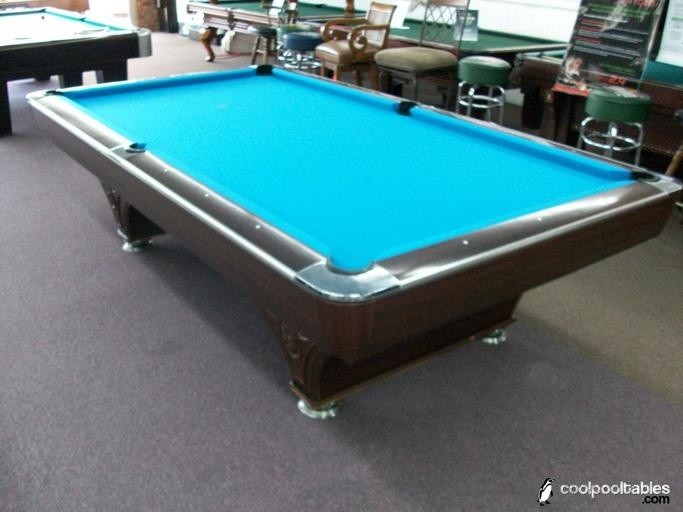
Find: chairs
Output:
[251,0,299,67]
[315,0,471,111]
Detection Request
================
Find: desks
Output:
[184,0,365,62]
[386,16,683,159]
[25,63,682,422]
[0,6,153,136]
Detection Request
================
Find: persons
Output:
[559,55,585,86]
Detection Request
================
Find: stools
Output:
[277,29,654,166]
